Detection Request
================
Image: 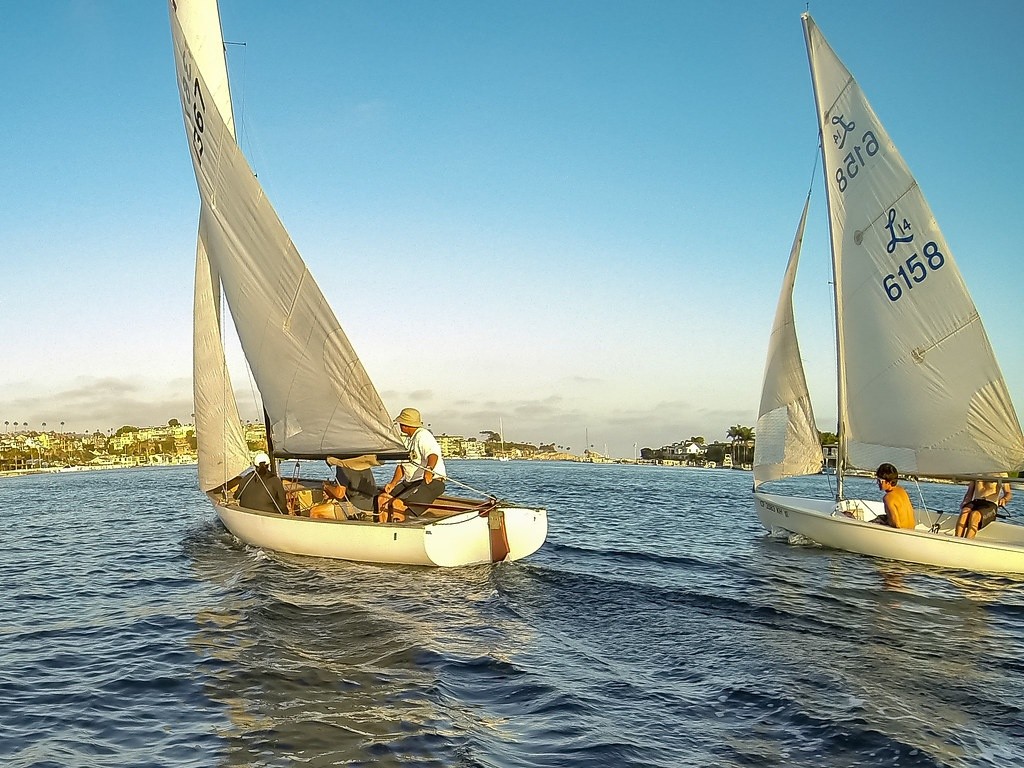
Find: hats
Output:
[254,453,270,466]
[395,408,424,427]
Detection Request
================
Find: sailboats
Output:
[167,1,548,568]
[750,11,1024,574]
[498,416,508,462]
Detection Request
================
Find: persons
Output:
[233,453,289,514]
[868,463,915,530]
[378,407,446,523]
[955,472,1012,539]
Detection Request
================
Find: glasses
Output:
[877,477,887,486]
